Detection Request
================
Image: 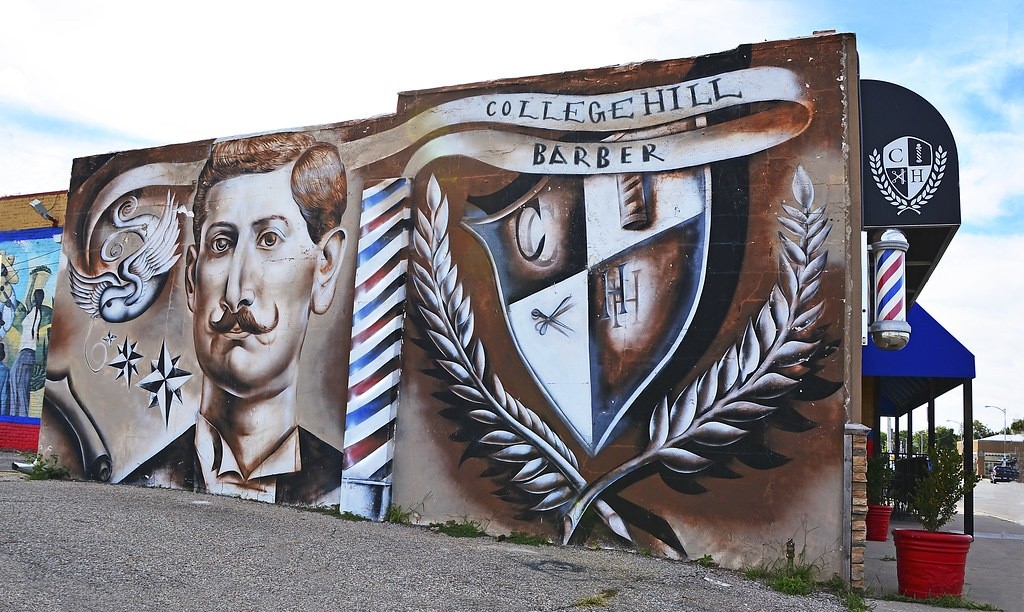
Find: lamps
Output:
[29,199,58,228]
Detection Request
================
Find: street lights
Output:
[985,405,1006,465]
[947,419,963,454]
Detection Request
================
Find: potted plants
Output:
[867,451,894,542]
[891,426,982,603]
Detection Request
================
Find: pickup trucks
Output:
[988,465,1015,483]
